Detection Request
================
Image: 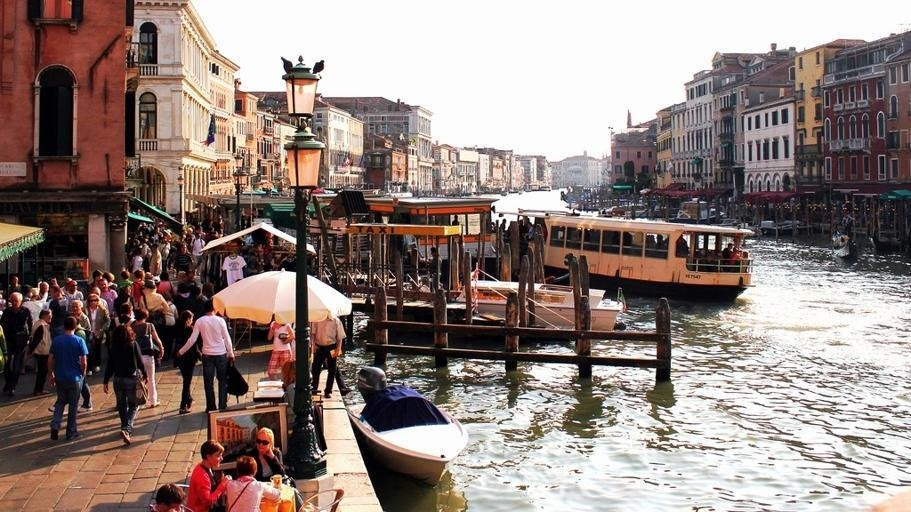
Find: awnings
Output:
[128,196,183,226]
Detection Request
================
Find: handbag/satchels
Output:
[139,323,160,355]
[133,367,149,405]
[218,357,248,396]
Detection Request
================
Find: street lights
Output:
[231,152,249,250]
[279,54,326,480]
[176,165,186,226]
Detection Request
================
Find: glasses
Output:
[256,439,269,445]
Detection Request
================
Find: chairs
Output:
[298,488,345,511]
[167,484,194,511]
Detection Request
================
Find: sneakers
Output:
[180,400,195,414]
[77,407,93,413]
[120,430,130,446]
[48,406,56,412]
[87,366,100,375]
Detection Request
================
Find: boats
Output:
[711,222,755,238]
[521,211,755,301]
[366,473,468,512]
[262,190,622,338]
[345,365,471,487]
[759,219,802,238]
[832,223,856,258]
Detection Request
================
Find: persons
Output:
[149,481,186,512]
[182,440,231,512]
[223,454,280,512]
[500,218,535,239]
[1,214,295,446]
[646,235,743,271]
[245,428,302,512]
[409,215,459,291]
[267,316,346,405]
[568,229,633,254]
[602,209,606,214]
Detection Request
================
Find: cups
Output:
[269,474,283,488]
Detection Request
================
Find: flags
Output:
[205,116,216,147]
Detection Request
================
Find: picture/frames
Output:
[208,402,288,470]
